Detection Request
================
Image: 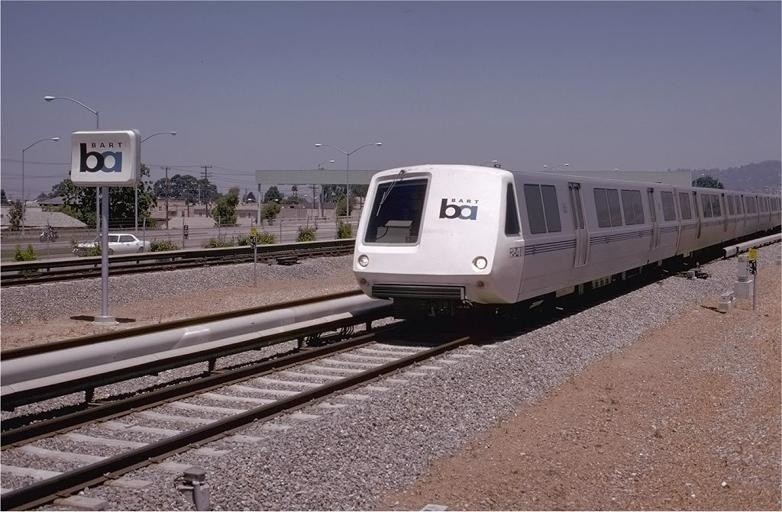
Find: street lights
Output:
[316,142,382,217]
[21,137,59,203]
[248,197,279,212]
[353,164,781,322]
[303,194,314,216]
[134,131,176,231]
[42,95,99,235]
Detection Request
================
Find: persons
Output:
[43,225,57,241]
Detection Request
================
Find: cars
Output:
[75,233,150,254]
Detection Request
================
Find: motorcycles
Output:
[39,227,58,243]
